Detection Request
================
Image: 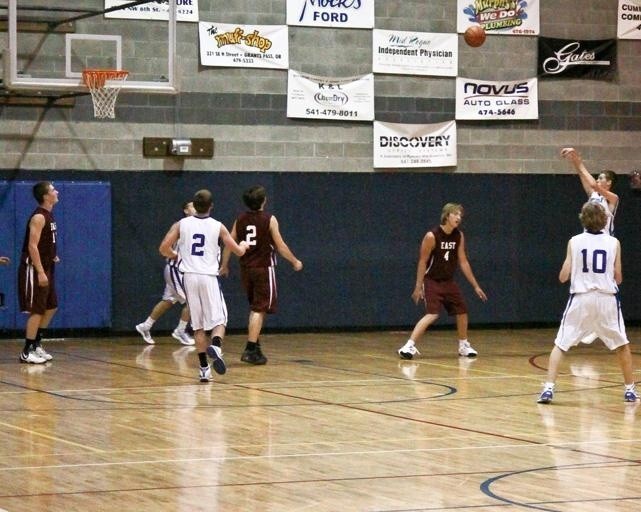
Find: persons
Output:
[218,185,304,365]
[158,188,250,383]
[538,202,639,404]
[16,181,60,365]
[135,200,196,346]
[397,202,488,360]
[561,147,621,238]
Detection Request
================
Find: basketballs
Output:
[465,27,487,48]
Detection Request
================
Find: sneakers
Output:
[20,351,48,364]
[625,387,641,402]
[199,364,215,382]
[136,323,157,345]
[241,348,268,365]
[398,343,418,359]
[457,342,479,357]
[35,346,54,360]
[207,344,227,375]
[171,328,196,346]
[536,384,556,403]
